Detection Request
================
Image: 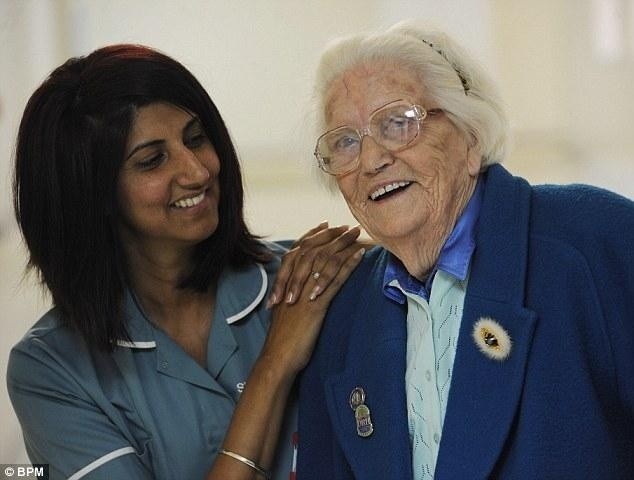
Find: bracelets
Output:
[216,447,271,478]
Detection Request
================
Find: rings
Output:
[309,270,323,281]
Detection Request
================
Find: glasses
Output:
[313,100,442,176]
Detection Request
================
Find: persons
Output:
[5,43,380,480]
[293,14,633,480]
